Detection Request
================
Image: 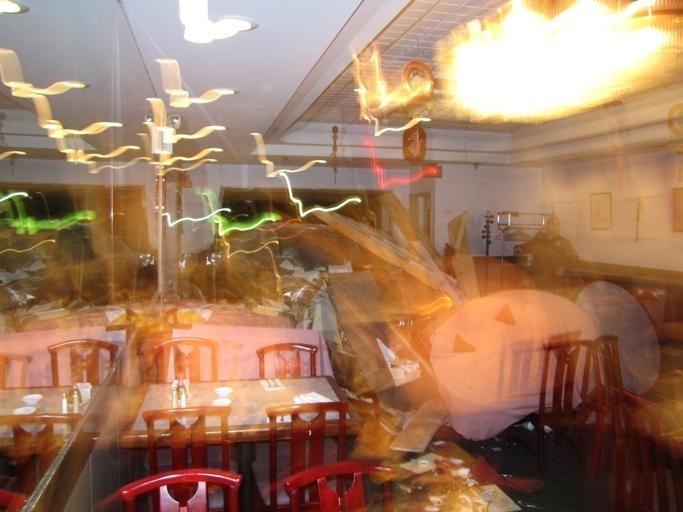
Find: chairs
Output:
[0,338,117,512]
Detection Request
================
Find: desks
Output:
[167,303,335,381]
[0,304,125,387]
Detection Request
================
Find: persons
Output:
[514,216,581,279]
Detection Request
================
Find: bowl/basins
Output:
[211,387,234,407]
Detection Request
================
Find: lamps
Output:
[402,121,427,166]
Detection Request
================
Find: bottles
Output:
[169,386,186,409]
[61,390,80,415]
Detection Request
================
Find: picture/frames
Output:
[590,192,611,230]
[673,187,683,232]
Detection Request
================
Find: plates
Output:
[12,394,44,415]
